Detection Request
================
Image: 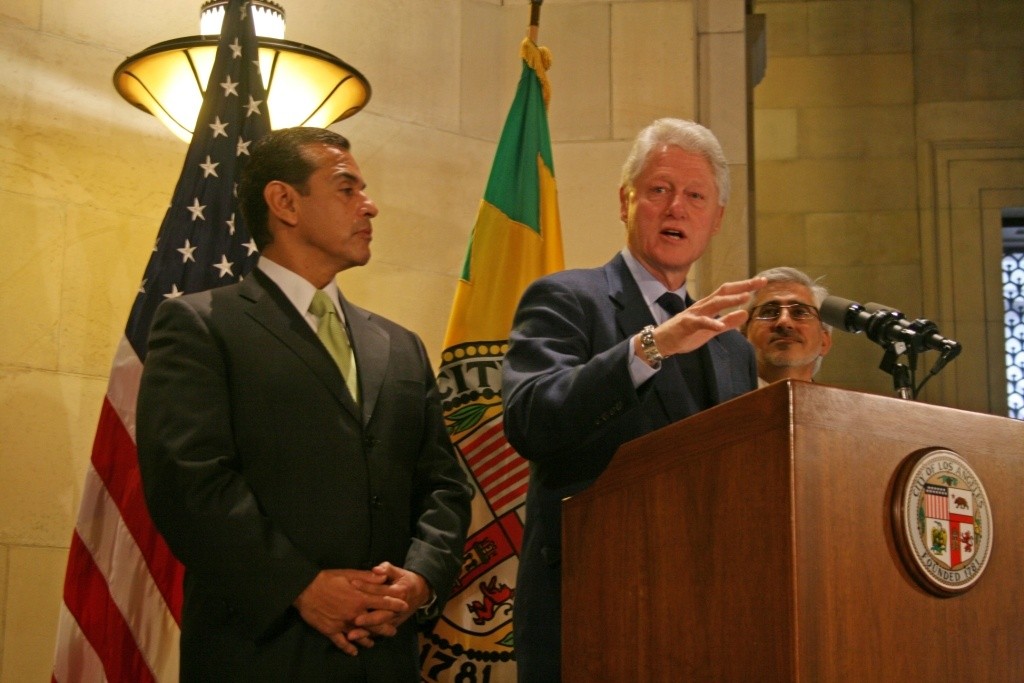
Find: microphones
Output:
[818,295,922,344]
[863,302,962,355]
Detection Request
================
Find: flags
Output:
[417,25,567,683]
[49,0,275,683]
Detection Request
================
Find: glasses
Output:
[743,303,825,333]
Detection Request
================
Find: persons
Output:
[135,127,473,683]
[739,267,834,391]
[500,119,769,683]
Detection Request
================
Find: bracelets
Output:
[639,325,665,365]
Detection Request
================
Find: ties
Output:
[308,289,360,406]
[655,291,709,411]
